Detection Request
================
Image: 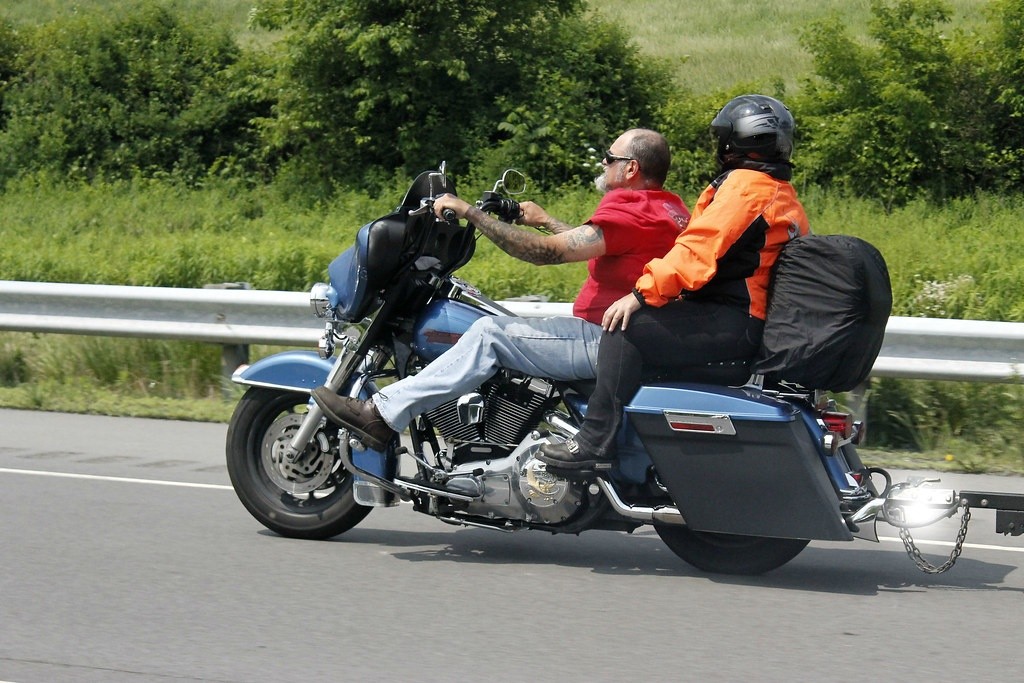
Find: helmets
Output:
[710,94,796,181]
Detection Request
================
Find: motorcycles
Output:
[224,160,881,577]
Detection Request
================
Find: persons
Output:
[536,94,809,473]
[310,128,691,452]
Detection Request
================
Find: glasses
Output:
[605,150,636,165]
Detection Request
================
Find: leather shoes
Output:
[310,386,395,454]
[535,437,619,471]
